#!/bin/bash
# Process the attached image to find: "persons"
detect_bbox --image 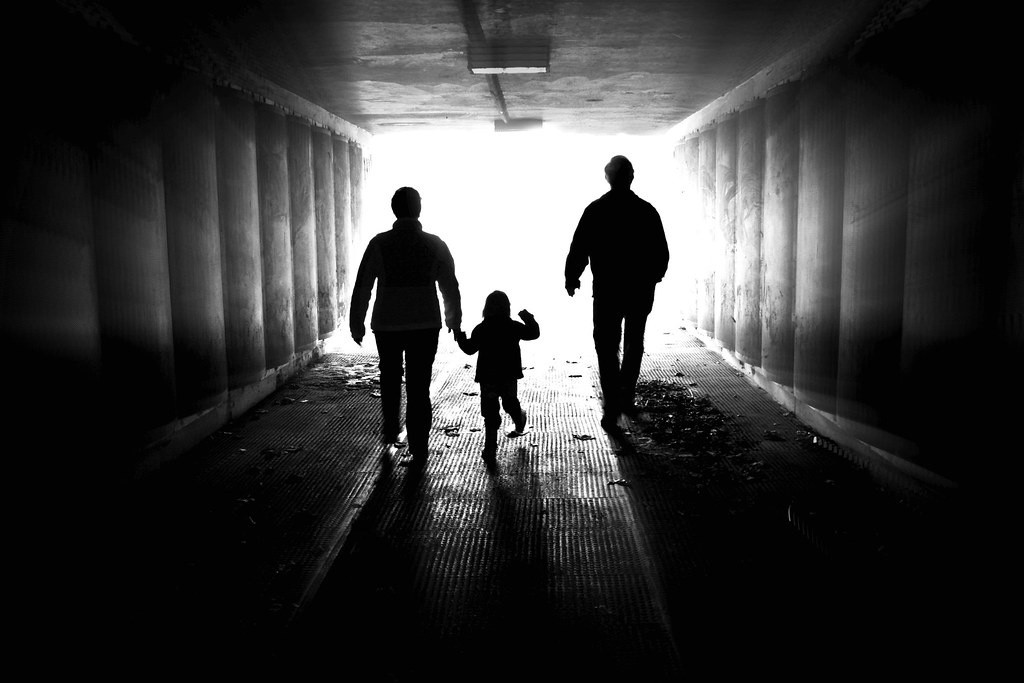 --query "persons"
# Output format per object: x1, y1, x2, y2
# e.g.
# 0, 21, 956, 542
564, 157, 670, 429
349, 187, 462, 462
455, 290, 540, 459
